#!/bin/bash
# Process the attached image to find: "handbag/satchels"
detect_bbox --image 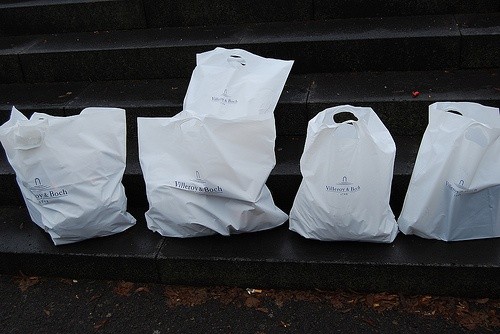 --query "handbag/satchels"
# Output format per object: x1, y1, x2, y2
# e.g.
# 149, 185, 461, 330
0, 105, 137, 246
289, 105, 398, 243
181, 47, 294, 130
137, 112, 288, 239
397, 102, 500, 242
